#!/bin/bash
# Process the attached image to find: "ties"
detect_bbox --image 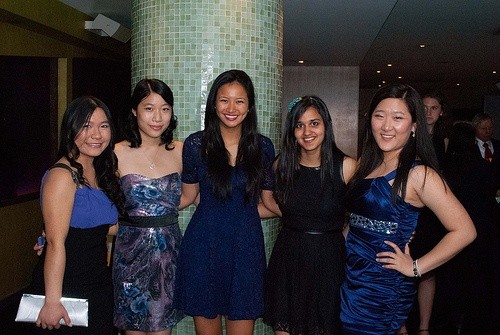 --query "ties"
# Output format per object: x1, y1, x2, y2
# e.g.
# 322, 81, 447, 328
483, 143, 493, 161
